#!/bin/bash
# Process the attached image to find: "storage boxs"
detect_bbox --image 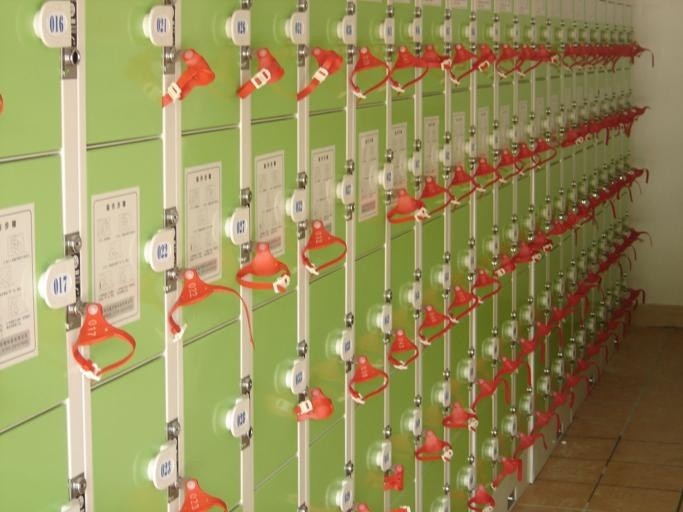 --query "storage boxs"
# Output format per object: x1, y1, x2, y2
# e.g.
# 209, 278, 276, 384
86, 142, 167, 389
93, 356, 170, 512
300, 1, 350, 113
76, 2, 168, 145
252, 118, 301, 309
173, 3, 241, 133
0, 405, 77, 512
3, 3, 63, 157
310, 271, 346, 443
254, 449, 297, 511
245, 0, 297, 121
255, 291, 296, 486
2, 153, 64, 433
309, 421, 352, 510
354, 1, 651, 510
185, 322, 240, 507
306, 108, 349, 283
185, 127, 244, 344
